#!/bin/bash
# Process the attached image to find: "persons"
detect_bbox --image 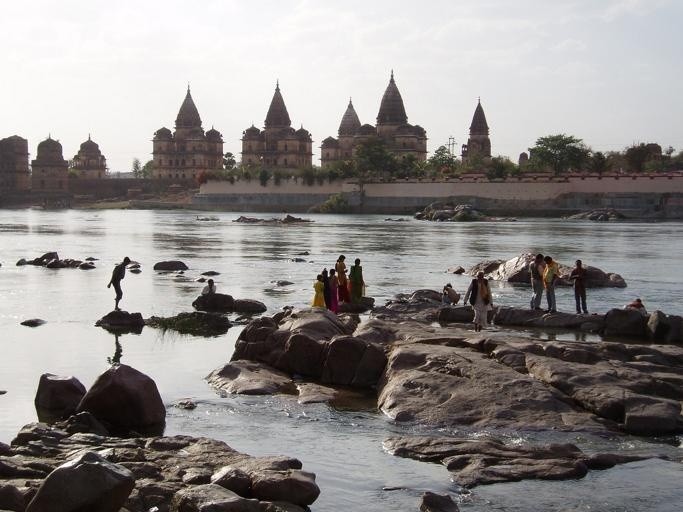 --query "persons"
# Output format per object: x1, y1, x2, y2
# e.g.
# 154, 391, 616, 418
312, 255, 365, 314
543, 256, 559, 312
528, 254, 545, 310
441, 283, 460, 305
570, 260, 589, 314
107, 257, 131, 311
463, 271, 493, 333
201, 279, 216, 296
624, 298, 647, 317
